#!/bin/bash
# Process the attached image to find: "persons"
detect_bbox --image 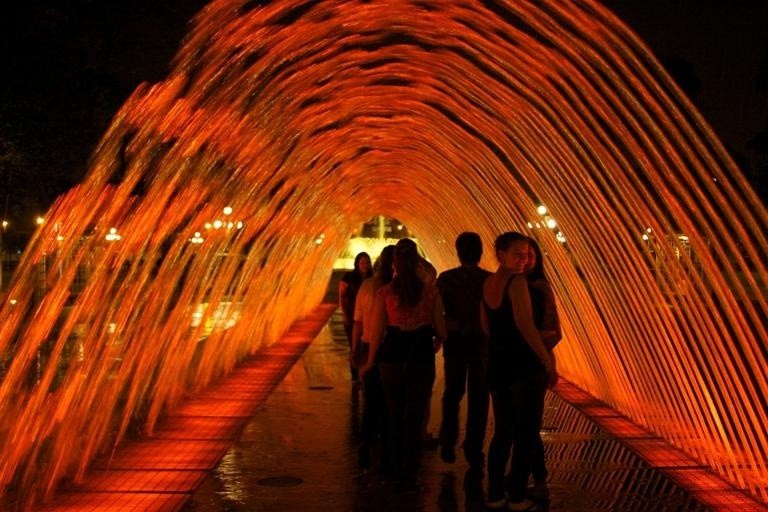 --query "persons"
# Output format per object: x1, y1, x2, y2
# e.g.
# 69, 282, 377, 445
338, 252, 370, 385
499, 235, 566, 504
354, 236, 436, 495
477, 233, 559, 512
431, 231, 496, 470
